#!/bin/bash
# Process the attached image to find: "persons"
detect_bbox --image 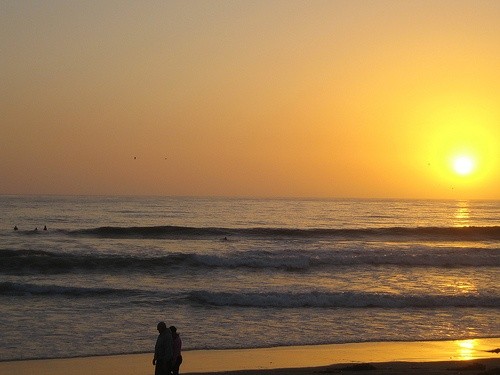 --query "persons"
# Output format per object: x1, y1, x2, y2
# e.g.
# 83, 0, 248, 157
44, 225, 47, 230
14, 226, 18, 230
35, 227, 38, 230
152, 317, 183, 375
